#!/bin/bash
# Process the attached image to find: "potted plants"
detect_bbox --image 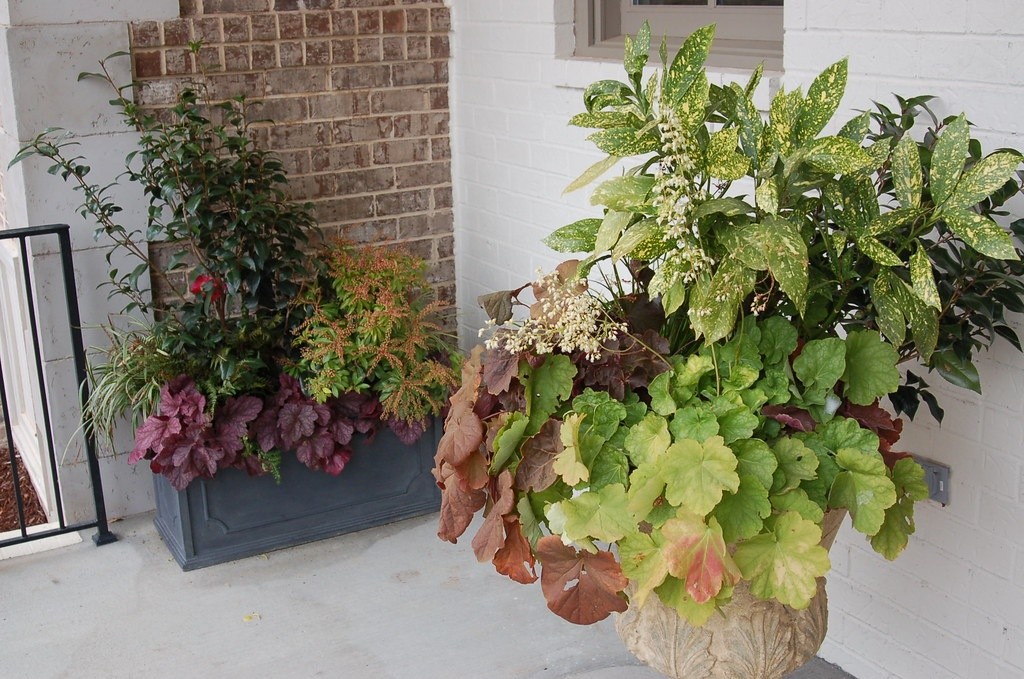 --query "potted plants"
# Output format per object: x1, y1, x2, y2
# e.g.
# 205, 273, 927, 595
6, 38, 456, 571
433, 21, 1024, 679
